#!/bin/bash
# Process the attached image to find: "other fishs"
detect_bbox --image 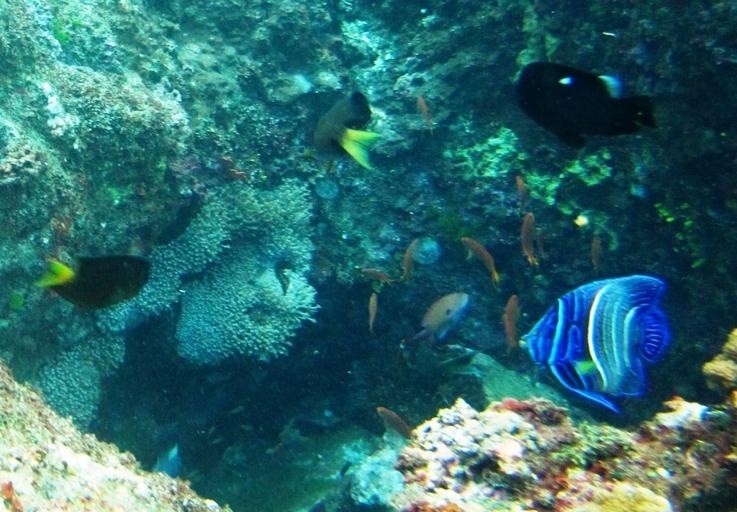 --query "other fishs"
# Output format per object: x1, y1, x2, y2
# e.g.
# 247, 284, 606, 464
359, 172, 604, 352
